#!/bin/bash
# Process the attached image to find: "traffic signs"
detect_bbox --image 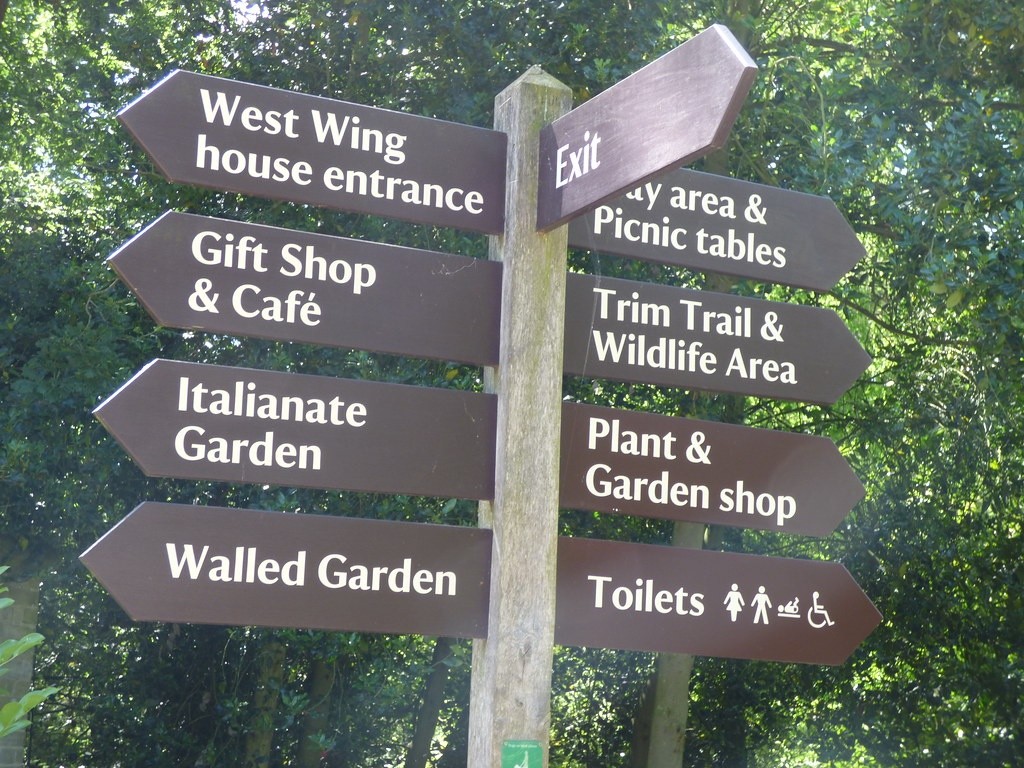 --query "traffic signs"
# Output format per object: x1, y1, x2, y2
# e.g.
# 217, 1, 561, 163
77, 18, 886, 670
